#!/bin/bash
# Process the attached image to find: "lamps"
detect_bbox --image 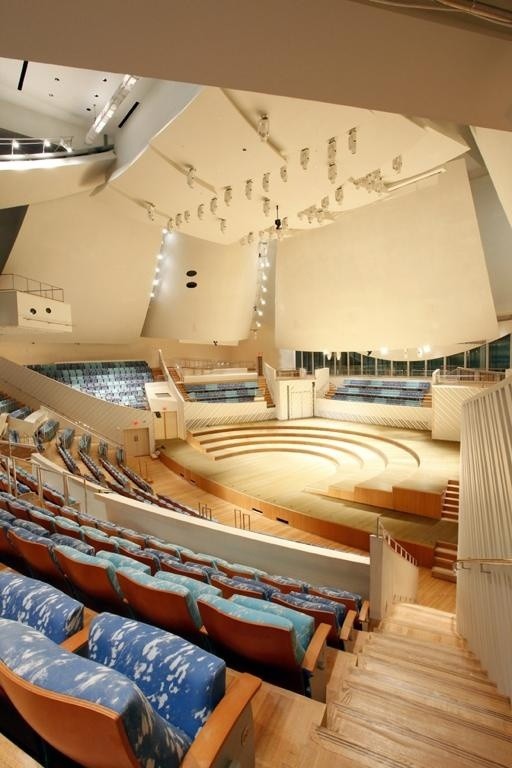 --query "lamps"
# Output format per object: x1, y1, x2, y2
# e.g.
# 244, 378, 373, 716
145, 115, 403, 244
83, 74, 140, 145
